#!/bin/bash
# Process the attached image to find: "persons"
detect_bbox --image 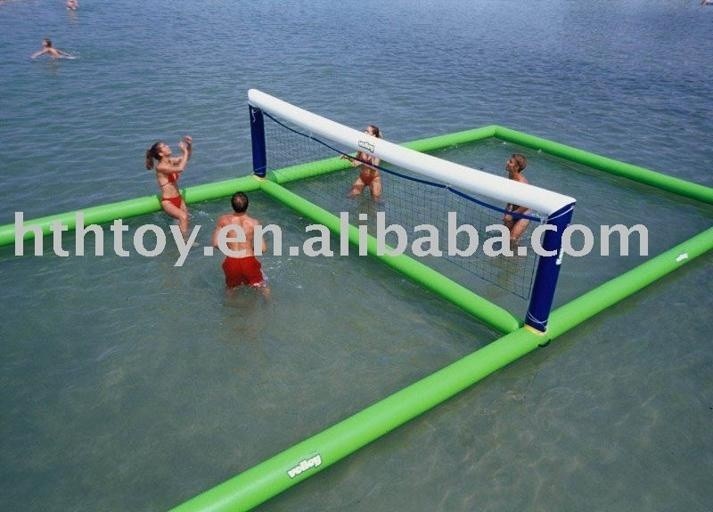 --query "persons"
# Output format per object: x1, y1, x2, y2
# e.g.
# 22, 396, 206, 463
145, 135, 192, 233
212, 192, 271, 301
33, 39, 75, 59
66, 0, 77, 10
503, 154, 531, 240
340, 125, 382, 202
